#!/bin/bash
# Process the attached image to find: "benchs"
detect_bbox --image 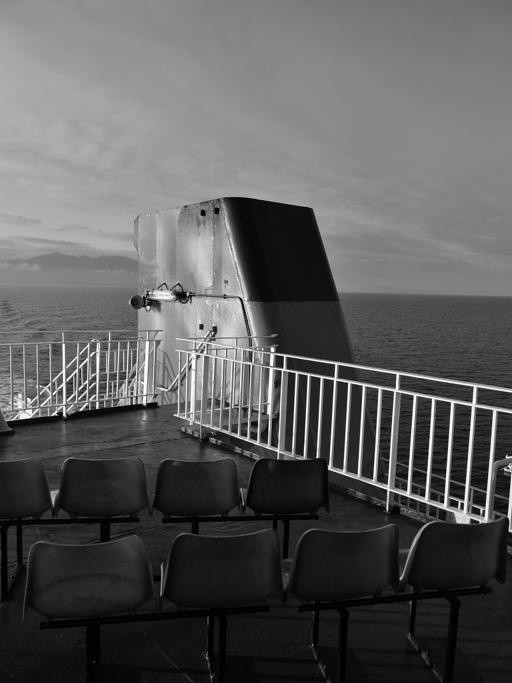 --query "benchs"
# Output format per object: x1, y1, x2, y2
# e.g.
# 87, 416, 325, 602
22, 517, 510, 682
1, 458, 330, 604
